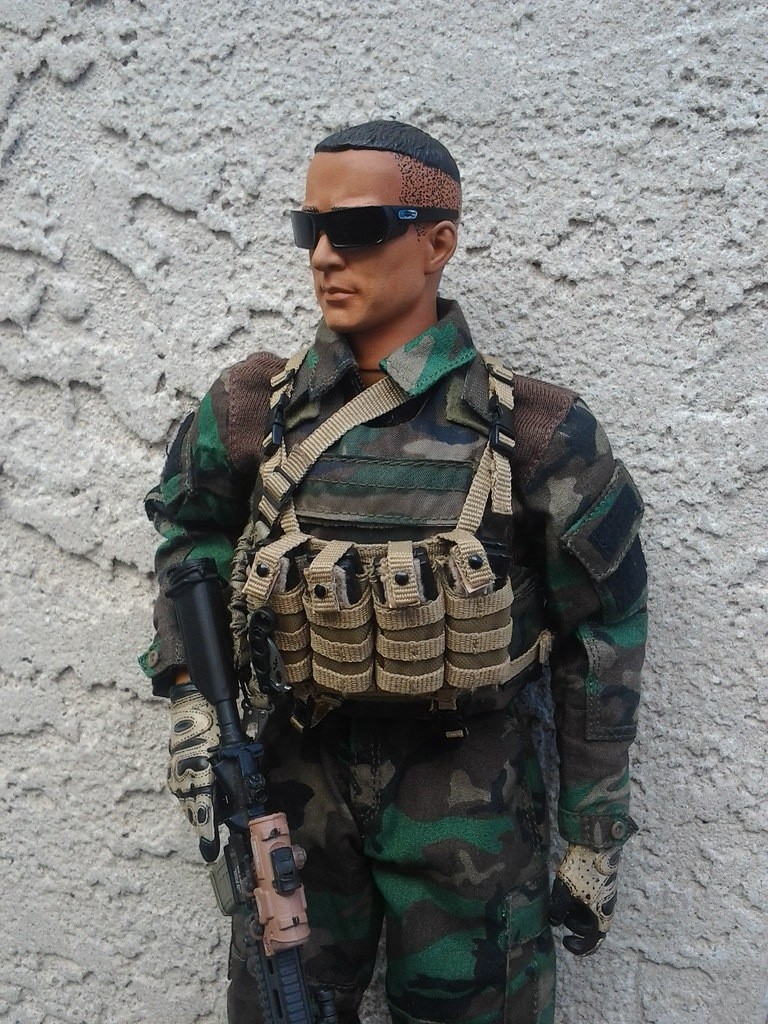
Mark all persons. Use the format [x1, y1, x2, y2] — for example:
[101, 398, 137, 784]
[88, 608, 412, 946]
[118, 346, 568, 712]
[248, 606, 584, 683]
[138, 117, 648, 1024]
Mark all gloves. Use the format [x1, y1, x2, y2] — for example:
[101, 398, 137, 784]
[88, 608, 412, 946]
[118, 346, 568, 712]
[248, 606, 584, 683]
[169, 681, 220, 826]
[549, 842, 619, 957]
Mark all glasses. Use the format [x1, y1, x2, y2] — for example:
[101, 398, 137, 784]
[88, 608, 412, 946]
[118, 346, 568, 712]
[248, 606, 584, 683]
[289, 205, 458, 249]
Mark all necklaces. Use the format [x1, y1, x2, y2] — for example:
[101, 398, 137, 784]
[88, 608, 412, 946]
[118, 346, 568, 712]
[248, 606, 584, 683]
[357, 366, 383, 372]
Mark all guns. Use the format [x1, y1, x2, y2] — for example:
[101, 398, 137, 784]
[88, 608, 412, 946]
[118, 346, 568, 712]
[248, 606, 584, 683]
[154, 556, 344, 1024]
